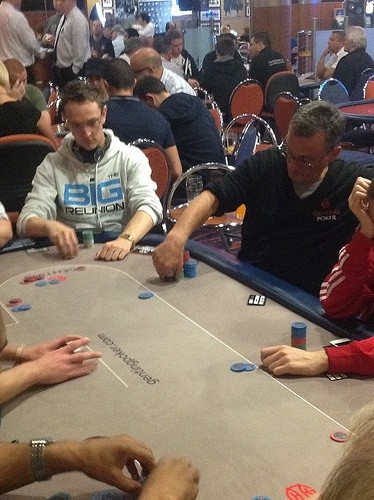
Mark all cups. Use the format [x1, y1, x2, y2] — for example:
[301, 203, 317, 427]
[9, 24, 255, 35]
[225, 134, 237, 153]
[236, 204, 246, 219]
[205, 94, 214, 109]
[292, 66, 297, 75]
[186, 175, 203, 206]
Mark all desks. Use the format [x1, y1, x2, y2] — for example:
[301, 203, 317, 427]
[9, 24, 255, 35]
[0, 232, 374, 500]
[335, 98, 374, 125]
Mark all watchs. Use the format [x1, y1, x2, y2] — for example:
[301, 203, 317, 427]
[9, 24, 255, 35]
[30, 437, 56, 481]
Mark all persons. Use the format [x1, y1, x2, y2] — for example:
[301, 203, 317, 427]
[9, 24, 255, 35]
[135, 77, 226, 197]
[16, 82, 163, 260]
[317, 31, 348, 79]
[249, 32, 287, 87]
[318, 403, 374, 500]
[0, 309, 102, 402]
[153, 103, 374, 297]
[240, 26, 249, 42]
[0, 201, 13, 248]
[99, 59, 182, 178]
[333, 26, 374, 96]
[196, 24, 247, 122]
[0, 0, 200, 146]
[261, 176, 374, 377]
[0, 434, 200, 500]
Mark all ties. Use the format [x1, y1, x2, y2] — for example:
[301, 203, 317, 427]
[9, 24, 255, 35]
[54, 15, 66, 63]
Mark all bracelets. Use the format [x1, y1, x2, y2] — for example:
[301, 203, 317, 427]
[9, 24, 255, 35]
[16, 344, 27, 360]
[119, 233, 135, 251]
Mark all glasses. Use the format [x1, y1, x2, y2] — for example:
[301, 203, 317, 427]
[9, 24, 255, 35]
[65, 111, 102, 133]
[277, 138, 335, 171]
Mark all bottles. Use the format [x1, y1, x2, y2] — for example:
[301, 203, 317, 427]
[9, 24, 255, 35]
[48, 86, 62, 125]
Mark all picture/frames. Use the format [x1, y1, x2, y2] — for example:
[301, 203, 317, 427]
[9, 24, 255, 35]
[198, 0, 221, 38]
[102, 0, 114, 17]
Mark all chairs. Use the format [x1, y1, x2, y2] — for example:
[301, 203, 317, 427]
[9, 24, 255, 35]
[0, 68, 374, 255]
